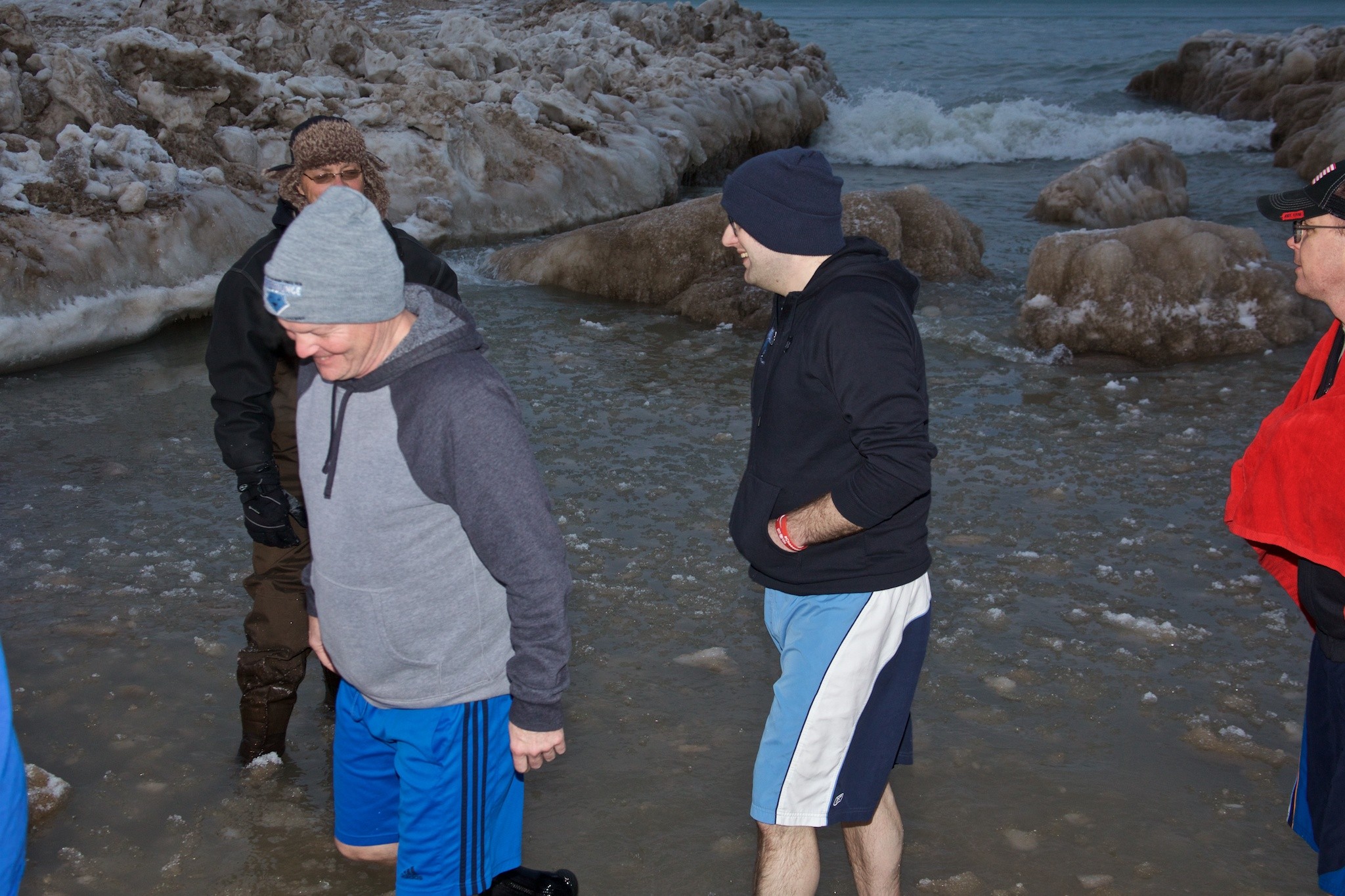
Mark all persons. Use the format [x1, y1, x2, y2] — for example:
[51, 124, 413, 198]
[1222, 158, 1345, 896]
[718, 146, 937, 896]
[205, 115, 462, 765]
[263, 182, 579, 896]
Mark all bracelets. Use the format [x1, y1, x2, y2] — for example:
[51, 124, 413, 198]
[776, 514, 808, 552]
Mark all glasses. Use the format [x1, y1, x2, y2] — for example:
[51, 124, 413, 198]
[728, 215, 741, 237]
[1293, 221, 1345, 244]
[300, 169, 363, 185]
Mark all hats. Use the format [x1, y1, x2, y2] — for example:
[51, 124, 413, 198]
[261, 115, 390, 223]
[1256, 160, 1345, 222]
[720, 146, 847, 256]
[261, 186, 404, 324]
